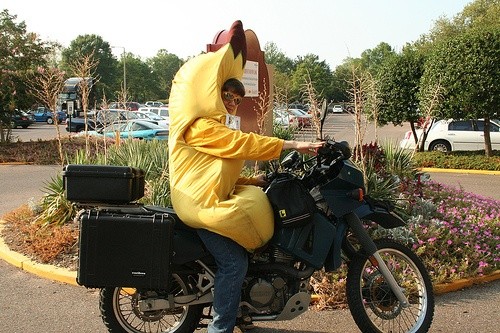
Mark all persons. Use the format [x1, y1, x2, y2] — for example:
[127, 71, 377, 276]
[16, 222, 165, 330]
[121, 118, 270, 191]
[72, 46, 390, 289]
[182, 77, 326, 333]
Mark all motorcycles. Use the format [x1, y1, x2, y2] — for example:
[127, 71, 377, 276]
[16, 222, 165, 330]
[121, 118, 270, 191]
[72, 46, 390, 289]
[60, 100, 436, 333]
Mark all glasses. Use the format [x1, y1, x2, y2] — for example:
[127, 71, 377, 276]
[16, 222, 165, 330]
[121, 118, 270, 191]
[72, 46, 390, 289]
[222, 93, 242, 105]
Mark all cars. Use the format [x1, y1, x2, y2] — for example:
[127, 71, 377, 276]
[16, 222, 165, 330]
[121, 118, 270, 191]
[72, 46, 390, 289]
[3, 77, 347, 140]
[397, 116, 500, 155]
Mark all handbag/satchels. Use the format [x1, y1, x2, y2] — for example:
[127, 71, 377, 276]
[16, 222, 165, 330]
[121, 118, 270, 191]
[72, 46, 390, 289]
[264, 171, 316, 228]
